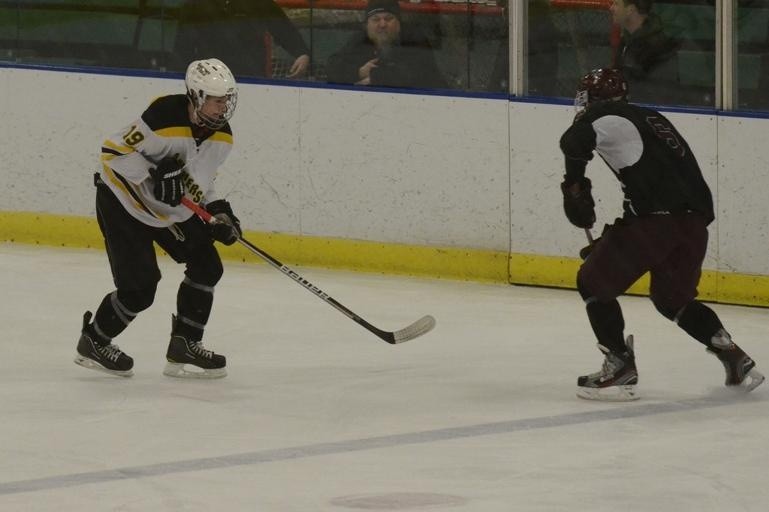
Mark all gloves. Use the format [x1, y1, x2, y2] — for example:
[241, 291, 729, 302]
[563, 175, 597, 226]
[207, 198, 241, 244]
[150, 157, 189, 204]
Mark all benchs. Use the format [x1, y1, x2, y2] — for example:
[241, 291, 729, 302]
[0, 0, 769, 105]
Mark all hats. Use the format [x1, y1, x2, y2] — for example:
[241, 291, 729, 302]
[361, 0, 403, 18]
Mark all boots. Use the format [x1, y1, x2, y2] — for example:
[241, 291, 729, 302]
[577, 336, 639, 388]
[711, 326, 757, 388]
[77, 313, 133, 370]
[167, 313, 226, 366]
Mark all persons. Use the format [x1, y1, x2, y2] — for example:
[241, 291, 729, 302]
[486, 0, 582, 100]
[325, 0, 449, 89]
[171, 0, 316, 83]
[559, 66, 766, 388]
[73, 58, 242, 372]
[609, 0, 698, 108]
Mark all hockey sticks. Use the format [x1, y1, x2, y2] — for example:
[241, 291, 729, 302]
[181, 196, 436, 345]
[584, 227, 633, 352]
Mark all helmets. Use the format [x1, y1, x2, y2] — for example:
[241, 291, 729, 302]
[573, 69, 628, 109]
[186, 57, 238, 129]
